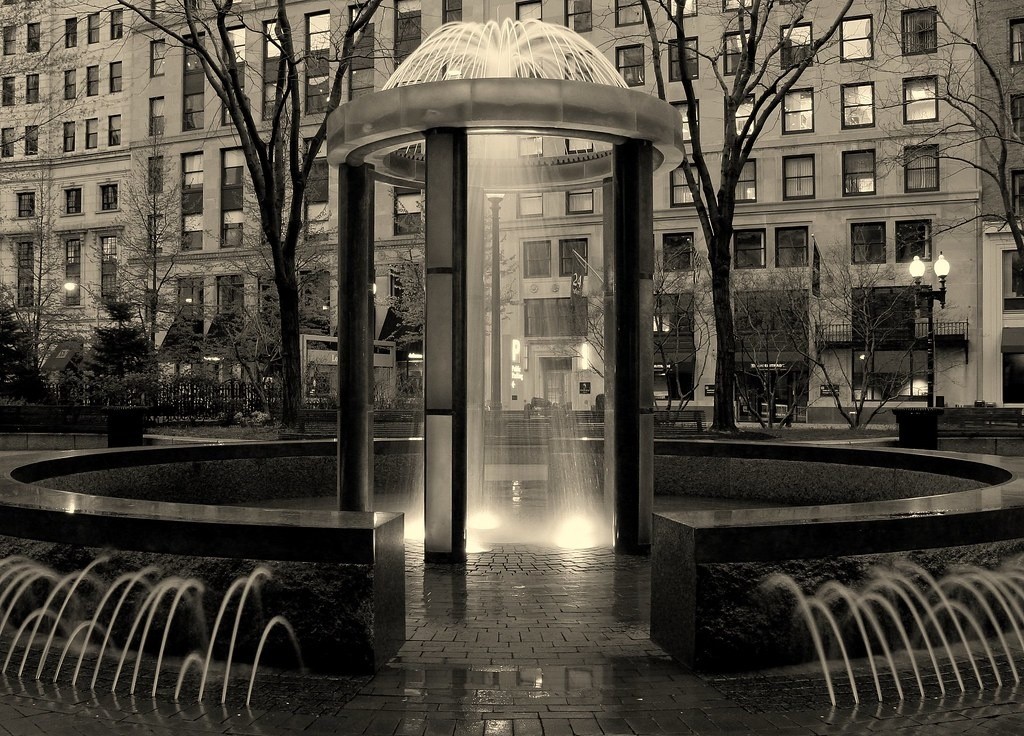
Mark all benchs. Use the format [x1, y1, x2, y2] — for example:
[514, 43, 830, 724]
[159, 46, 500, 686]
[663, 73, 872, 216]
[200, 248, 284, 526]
[939, 407, 1024, 455]
[484, 408, 557, 442]
[284, 408, 416, 445]
[655, 411, 713, 438]
[565, 409, 604, 440]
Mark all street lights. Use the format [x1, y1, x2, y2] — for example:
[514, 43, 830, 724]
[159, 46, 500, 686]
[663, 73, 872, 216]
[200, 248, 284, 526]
[910, 251, 950, 406]
